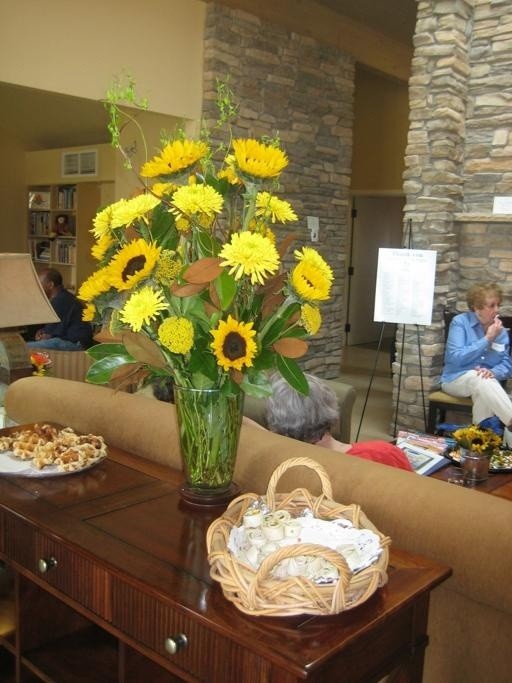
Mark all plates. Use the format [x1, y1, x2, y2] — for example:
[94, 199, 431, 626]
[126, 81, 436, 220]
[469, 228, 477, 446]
[0, 451, 108, 477]
[445, 447, 512, 474]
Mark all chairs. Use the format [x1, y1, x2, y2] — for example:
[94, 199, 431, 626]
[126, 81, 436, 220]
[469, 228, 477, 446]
[426, 311, 512, 435]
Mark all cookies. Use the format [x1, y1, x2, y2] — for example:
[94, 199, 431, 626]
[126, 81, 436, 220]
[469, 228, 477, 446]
[0, 423, 109, 473]
[242, 507, 359, 580]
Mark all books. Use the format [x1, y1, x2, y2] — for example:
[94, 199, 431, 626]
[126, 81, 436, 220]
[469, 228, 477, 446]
[25, 186, 76, 262]
[392, 429, 457, 475]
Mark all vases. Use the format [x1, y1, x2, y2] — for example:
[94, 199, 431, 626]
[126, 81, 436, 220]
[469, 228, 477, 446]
[173, 385, 245, 515]
[464, 454, 484, 481]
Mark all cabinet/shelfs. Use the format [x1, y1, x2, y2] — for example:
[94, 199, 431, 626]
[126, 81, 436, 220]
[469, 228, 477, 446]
[24, 181, 99, 297]
[0, 419, 456, 683]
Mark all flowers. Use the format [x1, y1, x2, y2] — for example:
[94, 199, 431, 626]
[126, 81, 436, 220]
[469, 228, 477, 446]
[78, 68, 339, 483]
[452, 423, 503, 457]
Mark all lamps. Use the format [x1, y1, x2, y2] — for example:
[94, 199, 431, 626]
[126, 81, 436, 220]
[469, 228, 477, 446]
[0, 252, 61, 384]
[355, 216, 437, 441]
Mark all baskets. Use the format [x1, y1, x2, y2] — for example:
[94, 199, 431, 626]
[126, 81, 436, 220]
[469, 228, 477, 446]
[206, 457, 392, 617]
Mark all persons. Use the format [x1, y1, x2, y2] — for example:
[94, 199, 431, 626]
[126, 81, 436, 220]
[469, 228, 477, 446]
[265, 370, 413, 471]
[23, 267, 95, 351]
[440, 279, 511, 435]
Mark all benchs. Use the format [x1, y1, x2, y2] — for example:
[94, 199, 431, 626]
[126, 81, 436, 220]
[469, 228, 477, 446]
[252, 368, 356, 442]
